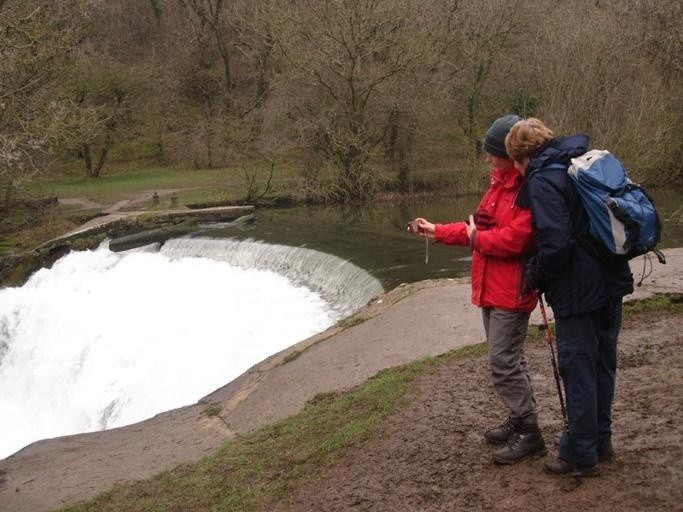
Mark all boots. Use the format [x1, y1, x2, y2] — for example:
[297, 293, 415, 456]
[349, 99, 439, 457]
[485, 414, 545, 464]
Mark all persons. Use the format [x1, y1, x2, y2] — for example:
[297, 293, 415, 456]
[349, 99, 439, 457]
[405, 114, 548, 466]
[505, 117, 635, 476]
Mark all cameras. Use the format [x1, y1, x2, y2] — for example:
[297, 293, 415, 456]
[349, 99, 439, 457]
[406, 220, 422, 233]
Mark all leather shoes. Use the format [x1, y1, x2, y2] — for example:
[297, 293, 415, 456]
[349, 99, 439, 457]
[544, 441, 613, 477]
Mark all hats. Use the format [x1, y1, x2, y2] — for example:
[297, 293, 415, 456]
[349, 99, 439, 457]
[483, 115, 522, 159]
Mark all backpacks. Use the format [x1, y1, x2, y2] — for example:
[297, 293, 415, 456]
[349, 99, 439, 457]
[528, 149, 667, 287]
